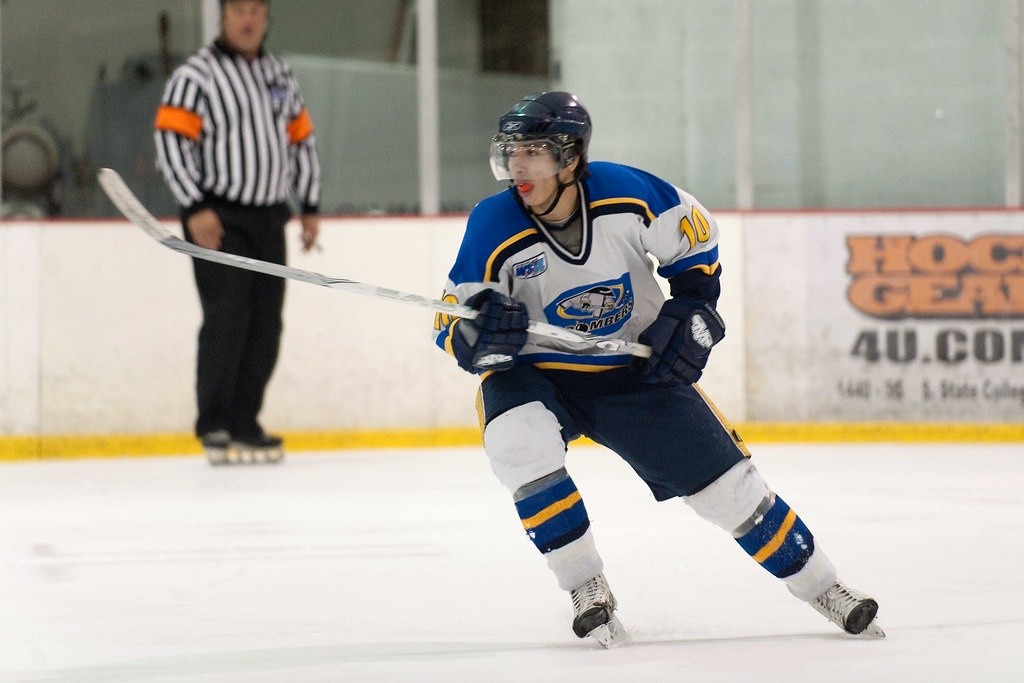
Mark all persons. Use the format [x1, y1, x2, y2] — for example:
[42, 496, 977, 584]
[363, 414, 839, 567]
[152, 0, 327, 453]
[431, 89, 880, 642]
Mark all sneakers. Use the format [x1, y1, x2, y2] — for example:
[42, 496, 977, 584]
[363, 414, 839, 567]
[569, 572, 632, 649]
[197, 421, 286, 466]
[812, 580, 887, 642]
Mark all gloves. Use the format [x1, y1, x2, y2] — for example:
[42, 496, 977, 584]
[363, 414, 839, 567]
[639, 297, 724, 389]
[451, 290, 528, 377]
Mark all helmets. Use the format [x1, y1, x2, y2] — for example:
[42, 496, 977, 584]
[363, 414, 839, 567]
[486, 91, 592, 184]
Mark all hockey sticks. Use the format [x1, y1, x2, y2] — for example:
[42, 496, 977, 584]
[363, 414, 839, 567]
[95, 166, 652, 358]
